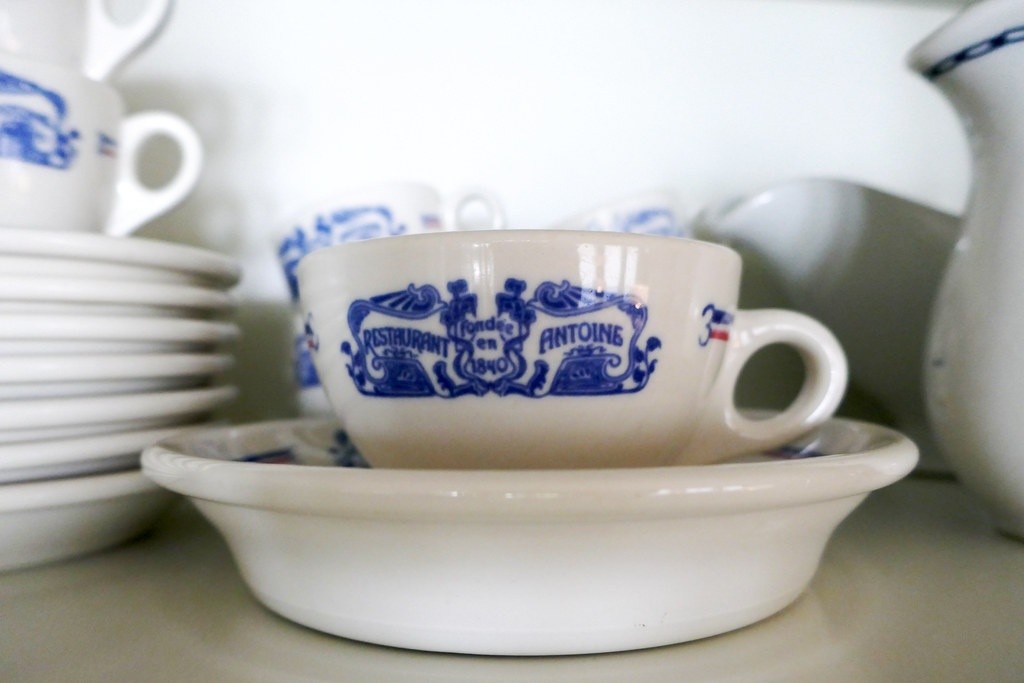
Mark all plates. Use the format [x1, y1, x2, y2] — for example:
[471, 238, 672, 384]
[0, 230, 243, 571]
[141, 411, 920, 658]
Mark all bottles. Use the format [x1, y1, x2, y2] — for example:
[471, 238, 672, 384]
[909, 0, 1023, 538]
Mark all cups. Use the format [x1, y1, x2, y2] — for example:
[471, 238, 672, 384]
[0, 58, 202, 237]
[279, 200, 508, 419]
[0, 1, 176, 82]
[299, 228, 847, 468]
